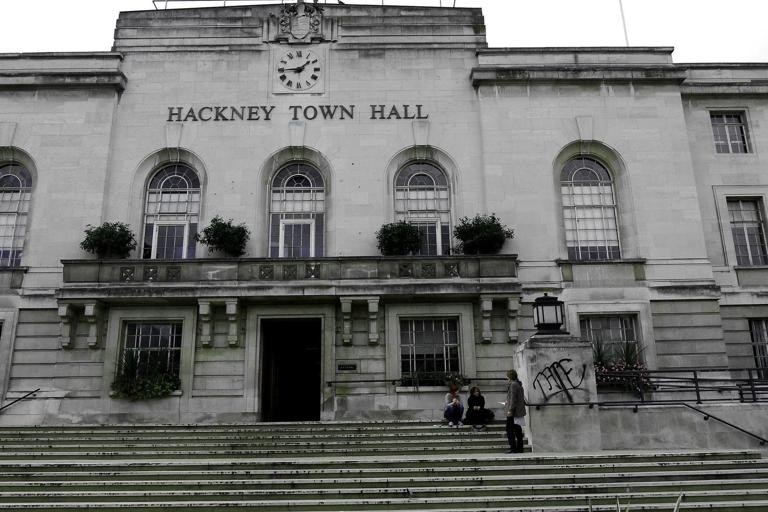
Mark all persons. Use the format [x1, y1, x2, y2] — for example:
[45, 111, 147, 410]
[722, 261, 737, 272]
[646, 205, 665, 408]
[443, 385, 485, 426]
[505, 369, 527, 453]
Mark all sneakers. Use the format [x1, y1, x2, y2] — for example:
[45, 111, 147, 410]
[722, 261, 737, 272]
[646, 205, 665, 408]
[449, 421, 464, 426]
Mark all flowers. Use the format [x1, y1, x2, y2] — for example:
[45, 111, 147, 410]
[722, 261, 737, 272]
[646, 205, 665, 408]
[593, 359, 654, 393]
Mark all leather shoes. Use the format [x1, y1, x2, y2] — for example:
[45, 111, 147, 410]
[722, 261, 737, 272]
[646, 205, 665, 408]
[505, 446, 523, 453]
